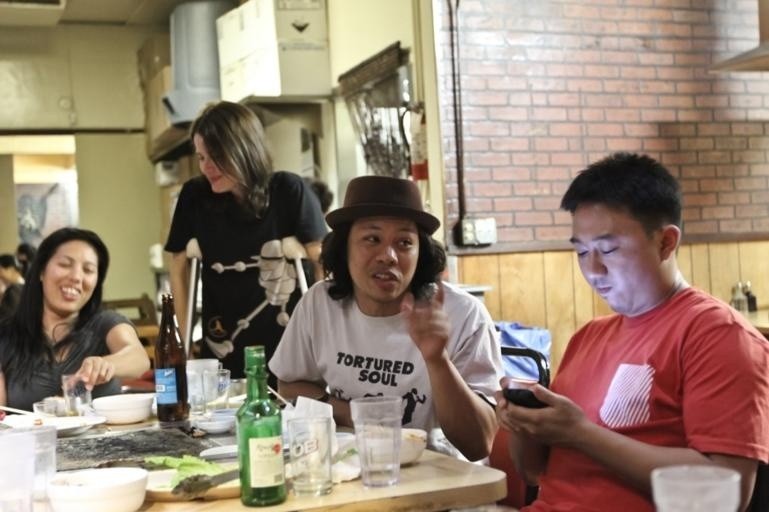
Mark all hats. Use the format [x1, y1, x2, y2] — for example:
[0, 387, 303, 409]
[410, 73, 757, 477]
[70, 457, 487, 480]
[325, 175, 440, 235]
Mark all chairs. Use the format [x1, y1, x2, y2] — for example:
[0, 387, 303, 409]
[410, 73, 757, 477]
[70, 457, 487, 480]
[104, 297, 158, 362]
[484, 346, 549, 511]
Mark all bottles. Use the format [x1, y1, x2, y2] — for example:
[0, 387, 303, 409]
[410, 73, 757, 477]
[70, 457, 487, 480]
[730, 281, 757, 313]
[154, 294, 190, 425]
[238, 344, 287, 507]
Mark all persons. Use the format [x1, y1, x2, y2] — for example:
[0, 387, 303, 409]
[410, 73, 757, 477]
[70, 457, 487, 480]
[0, 242, 36, 314]
[267, 174, 503, 461]
[498, 155, 768, 511]
[164, 102, 330, 399]
[0, 228, 152, 415]
[302, 181, 332, 284]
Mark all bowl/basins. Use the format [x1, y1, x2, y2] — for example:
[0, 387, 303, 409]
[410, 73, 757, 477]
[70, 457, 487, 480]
[93, 394, 154, 426]
[374, 428, 428, 467]
[43, 466, 149, 512]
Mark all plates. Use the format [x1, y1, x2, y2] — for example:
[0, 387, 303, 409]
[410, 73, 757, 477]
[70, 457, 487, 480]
[3, 412, 106, 437]
[144, 461, 241, 501]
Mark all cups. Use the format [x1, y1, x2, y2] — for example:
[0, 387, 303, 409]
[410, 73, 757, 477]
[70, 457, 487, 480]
[650, 464, 742, 511]
[0, 424, 58, 512]
[185, 358, 403, 497]
[32, 374, 92, 416]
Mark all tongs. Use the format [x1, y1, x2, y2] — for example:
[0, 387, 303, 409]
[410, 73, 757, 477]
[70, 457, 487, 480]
[96, 448, 291, 501]
